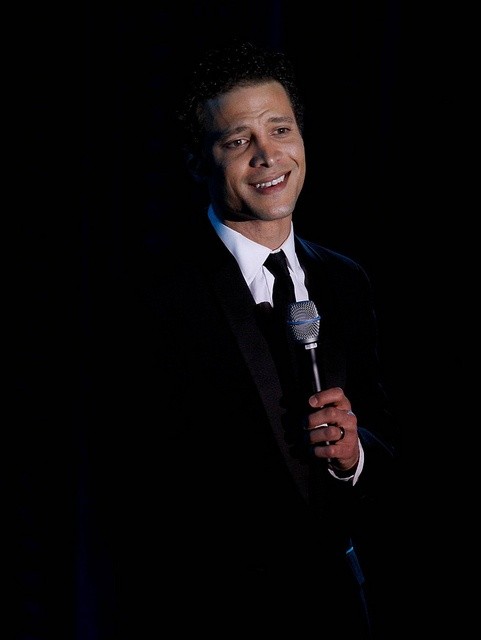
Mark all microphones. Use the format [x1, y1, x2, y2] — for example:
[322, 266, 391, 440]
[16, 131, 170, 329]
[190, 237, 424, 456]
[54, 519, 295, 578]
[287, 299, 336, 448]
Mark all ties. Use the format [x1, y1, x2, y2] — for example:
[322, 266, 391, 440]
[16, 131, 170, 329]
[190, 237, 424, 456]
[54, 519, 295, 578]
[263, 252, 308, 405]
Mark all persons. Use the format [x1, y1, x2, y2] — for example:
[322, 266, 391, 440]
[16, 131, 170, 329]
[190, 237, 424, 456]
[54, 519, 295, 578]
[128, 40, 418, 590]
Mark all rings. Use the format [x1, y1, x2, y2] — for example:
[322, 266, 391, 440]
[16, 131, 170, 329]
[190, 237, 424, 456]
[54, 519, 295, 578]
[337, 426, 346, 443]
